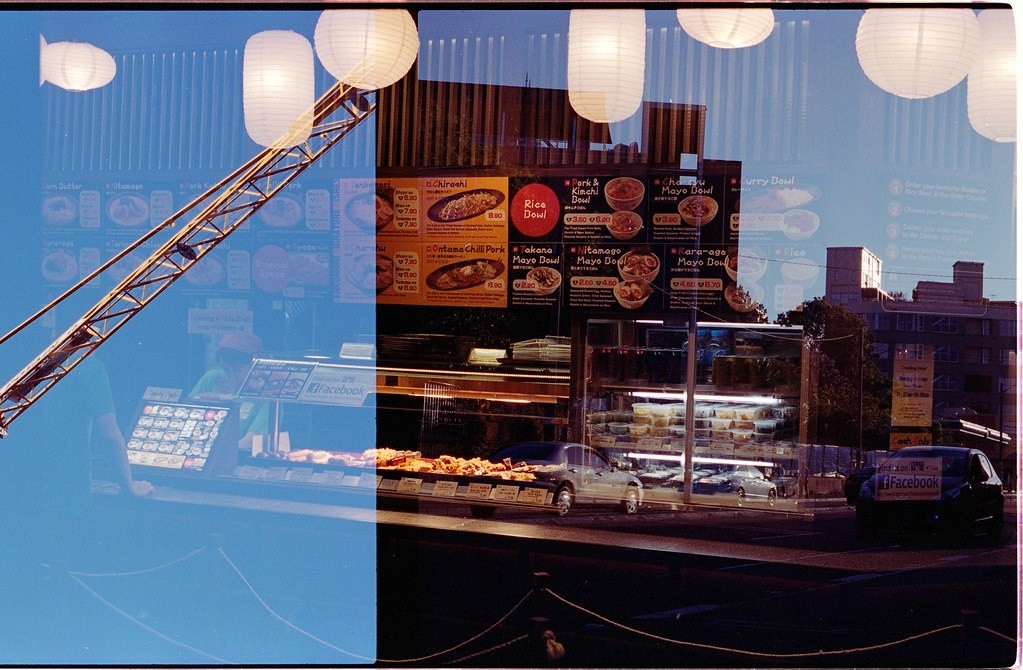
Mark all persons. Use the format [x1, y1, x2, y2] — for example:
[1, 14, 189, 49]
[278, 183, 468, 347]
[179, 332, 287, 461]
[26, 305, 155, 606]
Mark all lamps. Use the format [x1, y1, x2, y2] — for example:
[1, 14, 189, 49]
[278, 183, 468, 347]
[855, 7, 976, 99]
[676, 7, 774, 50]
[965, 9, 1016, 144]
[567, 8, 648, 123]
[38, 33, 116, 94]
[241, 29, 316, 150]
[313, 9, 421, 92]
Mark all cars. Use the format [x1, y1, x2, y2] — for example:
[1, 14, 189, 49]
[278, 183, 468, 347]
[492, 441, 643, 514]
[856, 445, 1003, 537]
[609, 452, 798, 508]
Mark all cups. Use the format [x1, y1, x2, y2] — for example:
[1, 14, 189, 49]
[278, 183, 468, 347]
[586, 344, 683, 388]
[586, 401, 798, 493]
[712, 355, 801, 391]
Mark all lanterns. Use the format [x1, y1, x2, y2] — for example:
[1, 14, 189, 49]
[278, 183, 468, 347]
[240, 29, 315, 151]
[674, 7, 777, 52]
[968, 8, 1016, 144]
[566, 7, 646, 125]
[38, 30, 51, 88]
[855, 6, 979, 99]
[310, 8, 418, 93]
[44, 39, 118, 96]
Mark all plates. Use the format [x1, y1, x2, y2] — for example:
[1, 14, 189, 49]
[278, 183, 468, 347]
[495, 357, 571, 366]
[351, 329, 480, 367]
[426, 258, 504, 291]
[426, 187, 505, 222]
[734, 184, 823, 212]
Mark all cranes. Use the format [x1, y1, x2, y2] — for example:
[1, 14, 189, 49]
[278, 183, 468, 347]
[0, 48, 376, 452]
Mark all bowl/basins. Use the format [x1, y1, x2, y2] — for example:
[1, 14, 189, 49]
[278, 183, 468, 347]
[613, 280, 652, 309]
[127, 406, 227, 467]
[38, 247, 81, 284]
[103, 251, 147, 285]
[345, 192, 399, 233]
[526, 267, 562, 294]
[344, 253, 398, 299]
[606, 212, 643, 240]
[244, 372, 305, 397]
[105, 192, 151, 230]
[724, 249, 767, 283]
[256, 192, 306, 231]
[38, 191, 80, 226]
[780, 258, 820, 289]
[179, 252, 227, 287]
[678, 196, 718, 226]
[605, 177, 644, 211]
[178, 193, 224, 230]
[725, 280, 763, 312]
[617, 251, 660, 284]
[779, 209, 821, 240]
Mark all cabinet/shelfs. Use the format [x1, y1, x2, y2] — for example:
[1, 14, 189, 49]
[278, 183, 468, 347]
[235, 355, 573, 516]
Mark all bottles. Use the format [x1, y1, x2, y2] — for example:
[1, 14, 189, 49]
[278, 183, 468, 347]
[733, 331, 763, 358]
[684, 329, 731, 386]
